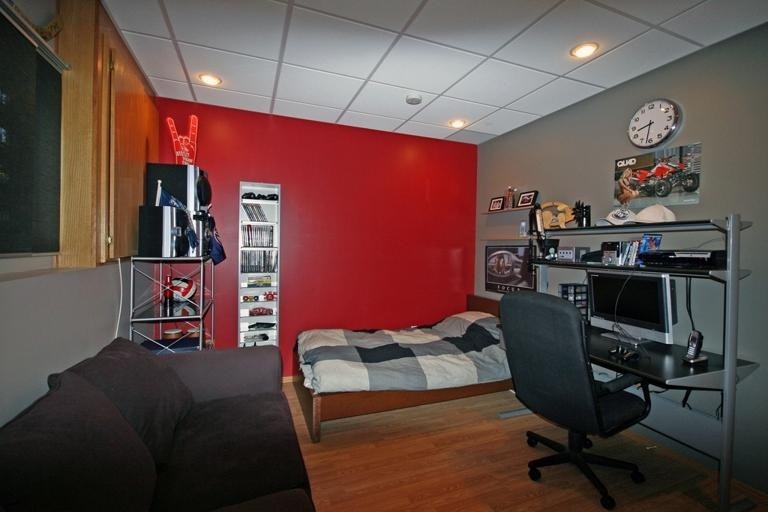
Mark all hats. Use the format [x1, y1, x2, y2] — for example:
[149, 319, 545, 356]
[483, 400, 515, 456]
[599, 208, 636, 226]
[622, 204, 676, 224]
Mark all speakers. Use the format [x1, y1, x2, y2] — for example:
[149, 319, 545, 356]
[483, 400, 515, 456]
[137, 205, 187, 258]
[144, 162, 211, 258]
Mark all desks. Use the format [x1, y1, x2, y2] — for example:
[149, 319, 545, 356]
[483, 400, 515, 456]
[499, 215, 758, 510]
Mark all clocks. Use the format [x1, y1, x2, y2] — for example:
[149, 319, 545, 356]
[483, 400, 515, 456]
[627, 97, 680, 149]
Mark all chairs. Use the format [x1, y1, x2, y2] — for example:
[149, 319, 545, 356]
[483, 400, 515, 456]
[501, 289, 653, 511]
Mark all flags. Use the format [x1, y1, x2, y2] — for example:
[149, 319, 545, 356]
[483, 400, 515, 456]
[153, 186, 199, 249]
[209, 218, 227, 266]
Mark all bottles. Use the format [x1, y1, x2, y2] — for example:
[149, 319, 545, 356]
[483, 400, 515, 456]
[163, 275, 174, 317]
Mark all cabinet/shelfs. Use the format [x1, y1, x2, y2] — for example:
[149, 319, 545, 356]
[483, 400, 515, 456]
[129, 256, 215, 353]
[236, 179, 281, 348]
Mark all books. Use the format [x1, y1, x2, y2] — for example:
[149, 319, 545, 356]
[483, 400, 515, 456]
[640, 233, 663, 252]
[241, 203, 279, 273]
[601, 241, 642, 266]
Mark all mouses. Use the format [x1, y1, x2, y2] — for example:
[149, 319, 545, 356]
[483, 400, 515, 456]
[609, 344, 625, 355]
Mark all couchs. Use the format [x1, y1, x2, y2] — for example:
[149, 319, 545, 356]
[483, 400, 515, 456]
[1, 344, 318, 511]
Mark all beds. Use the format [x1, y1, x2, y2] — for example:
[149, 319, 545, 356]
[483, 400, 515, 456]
[292, 292, 514, 443]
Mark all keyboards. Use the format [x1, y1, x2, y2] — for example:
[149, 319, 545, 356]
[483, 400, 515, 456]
[591, 363, 624, 384]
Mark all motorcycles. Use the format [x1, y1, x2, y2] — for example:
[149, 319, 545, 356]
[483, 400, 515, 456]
[626, 153, 700, 196]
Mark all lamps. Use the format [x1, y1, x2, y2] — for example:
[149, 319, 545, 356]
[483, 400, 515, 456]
[525, 202, 547, 275]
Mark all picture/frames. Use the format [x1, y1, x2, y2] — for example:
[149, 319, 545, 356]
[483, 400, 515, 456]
[488, 190, 539, 212]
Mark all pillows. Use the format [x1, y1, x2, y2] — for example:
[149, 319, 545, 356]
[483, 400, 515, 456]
[1, 336, 196, 511]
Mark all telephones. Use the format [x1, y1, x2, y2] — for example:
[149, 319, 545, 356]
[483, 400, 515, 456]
[681, 330, 708, 364]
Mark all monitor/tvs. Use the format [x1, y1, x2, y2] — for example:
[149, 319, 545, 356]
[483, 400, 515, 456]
[586, 268, 678, 347]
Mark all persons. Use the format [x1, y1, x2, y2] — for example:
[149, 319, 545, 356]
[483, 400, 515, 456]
[493, 255, 507, 275]
[618, 168, 640, 207]
[649, 236, 657, 250]
